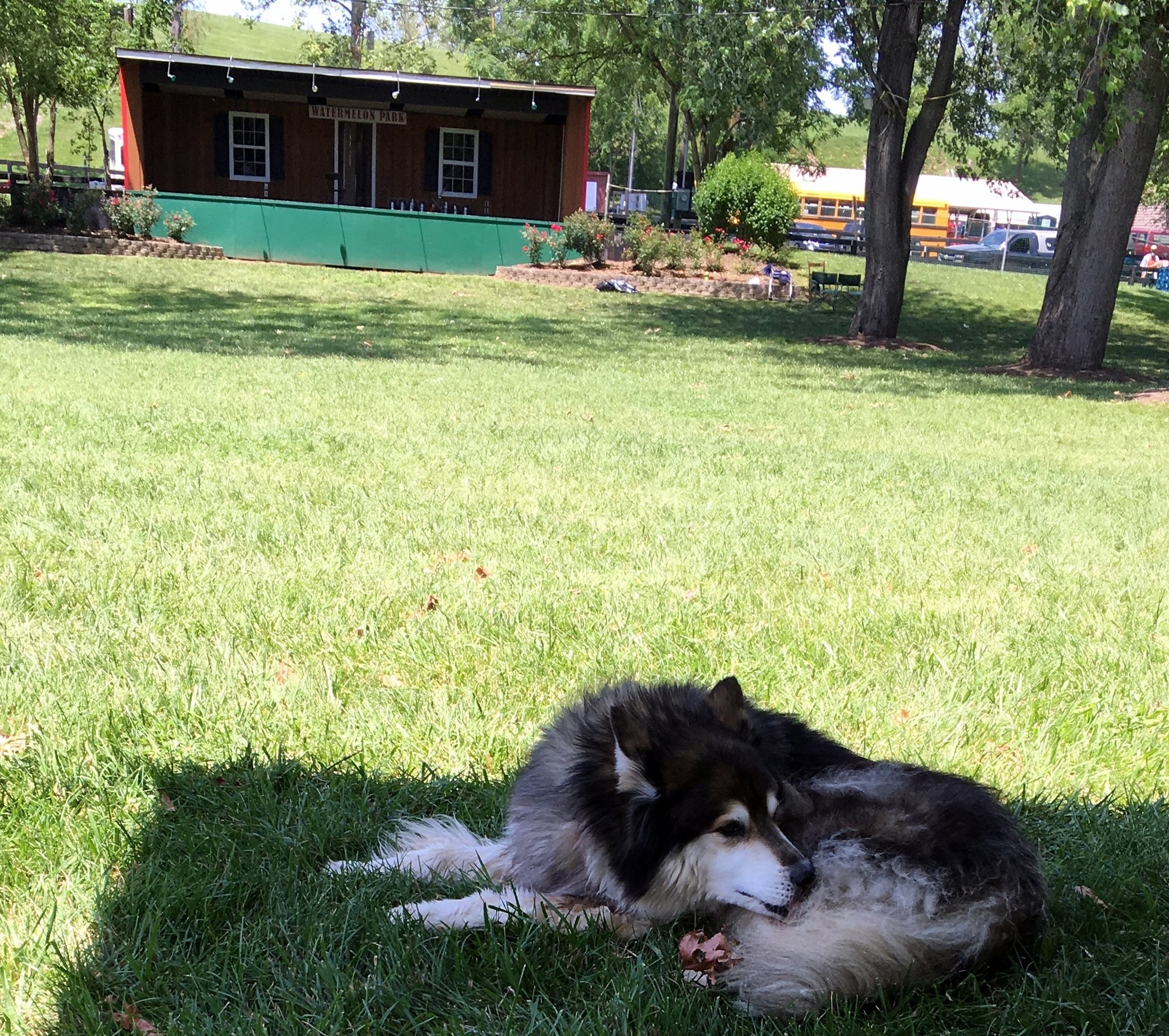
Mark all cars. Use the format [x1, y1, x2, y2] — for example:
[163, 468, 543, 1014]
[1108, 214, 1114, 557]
[782, 222, 839, 252]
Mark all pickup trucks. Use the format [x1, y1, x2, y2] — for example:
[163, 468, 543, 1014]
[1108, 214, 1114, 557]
[937, 230, 1059, 268]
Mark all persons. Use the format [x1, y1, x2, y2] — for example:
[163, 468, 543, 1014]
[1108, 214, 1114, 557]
[960, 226, 965, 235]
[1140, 245, 1159, 288]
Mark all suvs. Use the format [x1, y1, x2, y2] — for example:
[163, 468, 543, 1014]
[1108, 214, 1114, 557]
[835, 219, 922, 256]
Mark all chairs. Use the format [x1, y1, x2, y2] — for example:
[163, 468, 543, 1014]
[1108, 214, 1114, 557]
[807, 260, 862, 313]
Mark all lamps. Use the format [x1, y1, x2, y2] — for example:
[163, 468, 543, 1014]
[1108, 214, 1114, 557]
[312, 63, 317, 92]
[531, 79, 538, 109]
[167, 53, 175, 81]
[392, 70, 400, 99]
[226, 55, 234, 82]
[475, 75, 481, 102]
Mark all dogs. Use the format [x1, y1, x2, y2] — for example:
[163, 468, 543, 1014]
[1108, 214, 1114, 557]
[320, 676, 1052, 1017]
